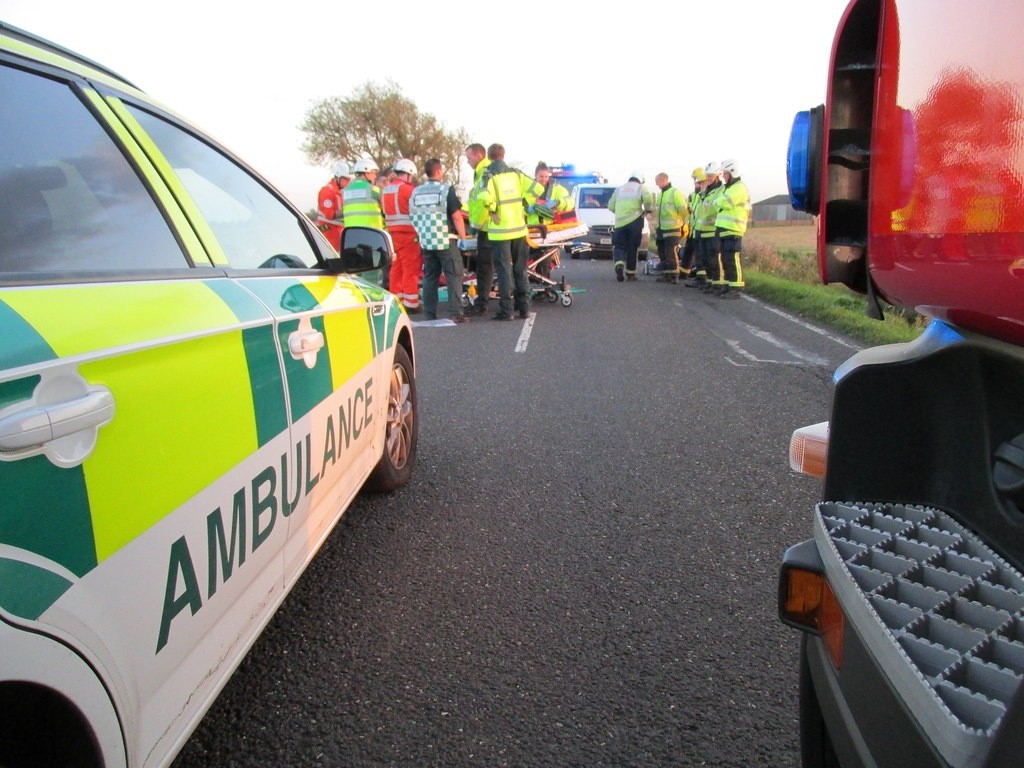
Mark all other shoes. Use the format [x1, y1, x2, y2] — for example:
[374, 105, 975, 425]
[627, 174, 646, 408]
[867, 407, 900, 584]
[628, 275, 637, 281]
[491, 312, 514, 321]
[405, 307, 421, 314]
[656, 273, 678, 283]
[679, 271, 743, 299]
[453, 315, 471, 323]
[520, 310, 531, 318]
[466, 305, 488, 316]
[615, 264, 624, 282]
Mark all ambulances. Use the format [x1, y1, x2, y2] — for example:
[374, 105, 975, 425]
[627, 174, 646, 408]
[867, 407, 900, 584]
[1, 25, 420, 768]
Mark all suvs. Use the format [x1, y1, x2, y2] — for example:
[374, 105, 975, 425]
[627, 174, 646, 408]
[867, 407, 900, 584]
[572, 185, 654, 262]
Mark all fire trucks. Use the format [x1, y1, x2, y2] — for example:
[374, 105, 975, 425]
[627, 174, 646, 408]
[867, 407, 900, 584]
[780, 2, 1024, 766]
[549, 163, 608, 203]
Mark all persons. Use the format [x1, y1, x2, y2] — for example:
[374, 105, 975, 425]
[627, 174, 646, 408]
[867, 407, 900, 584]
[318, 160, 422, 314]
[464, 144, 575, 321]
[408, 158, 470, 323]
[584, 195, 600, 206]
[608, 160, 751, 299]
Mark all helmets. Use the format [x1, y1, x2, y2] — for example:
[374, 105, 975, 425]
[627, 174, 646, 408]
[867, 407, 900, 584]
[695, 175, 706, 182]
[334, 162, 353, 178]
[353, 158, 380, 173]
[720, 159, 742, 178]
[704, 162, 722, 175]
[394, 159, 417, 176]
[692, 168, 703, 177]
[628, 174, 646, 184]
